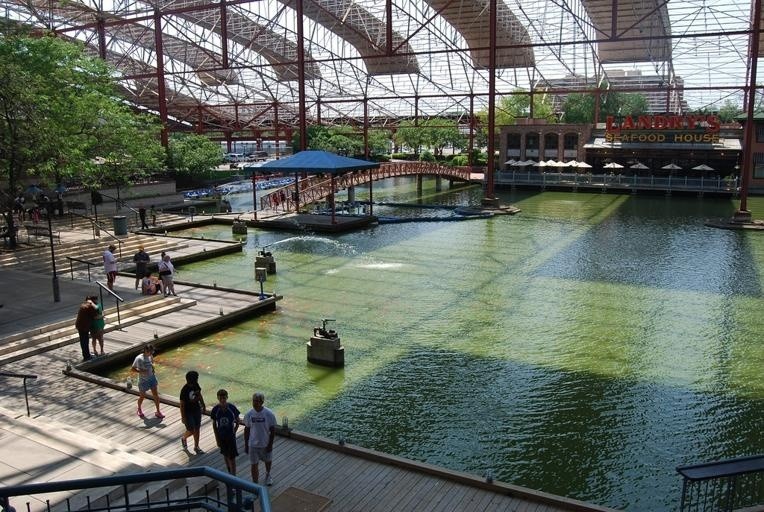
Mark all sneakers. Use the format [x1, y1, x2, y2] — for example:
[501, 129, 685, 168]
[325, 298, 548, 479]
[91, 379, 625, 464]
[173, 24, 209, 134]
[264, 472, 274, 486]
[155, 411, 165, 419]
[193, 446, 204, 454]
[137, 410, 144, 417]
[181, 436, 187, 450]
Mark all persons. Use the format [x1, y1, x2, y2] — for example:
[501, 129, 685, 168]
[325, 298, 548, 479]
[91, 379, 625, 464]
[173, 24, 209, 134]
[241, 393, 277, 487]
[91, 295, 104, 356]
[210, 389, 241, 484]
[13, 185, 67, 225]
[132, 246, 178, 297]
[74, 295, 105, 361]
[272, 192, 278, 211]
[149, 204, 157, 225]
[279, 190, 286, 210]
[179, 371, 206, 454]
[139, 203, 147, 229]
[102, 244, 119, 294]
[129, 344, 166, 419]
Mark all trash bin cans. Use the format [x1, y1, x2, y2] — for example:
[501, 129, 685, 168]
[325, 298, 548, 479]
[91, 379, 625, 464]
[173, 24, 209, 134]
[114, 216, 128, 234]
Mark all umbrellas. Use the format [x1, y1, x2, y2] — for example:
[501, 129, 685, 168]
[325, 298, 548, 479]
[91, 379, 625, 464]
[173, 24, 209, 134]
[503, 156, 716, 174]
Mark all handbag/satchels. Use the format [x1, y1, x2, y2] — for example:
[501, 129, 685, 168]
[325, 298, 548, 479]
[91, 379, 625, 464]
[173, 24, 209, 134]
[159, 263, 172, 277]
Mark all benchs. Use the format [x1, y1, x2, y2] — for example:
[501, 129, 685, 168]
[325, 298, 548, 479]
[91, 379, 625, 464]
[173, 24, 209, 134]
[24, 224, 62, 245]
